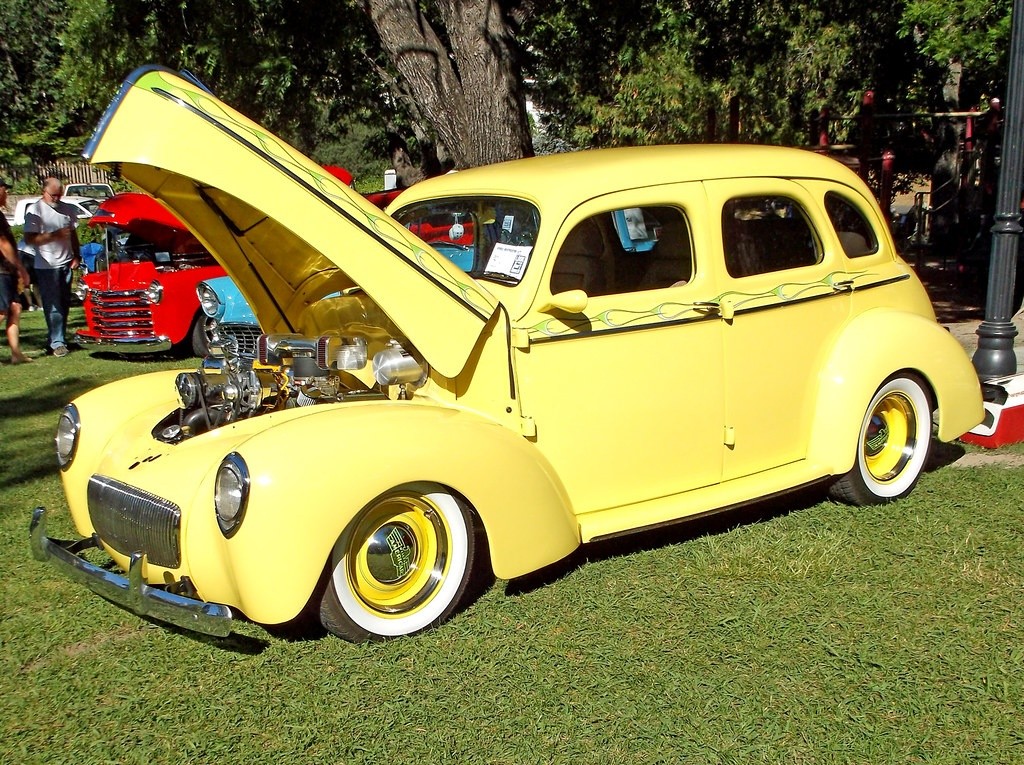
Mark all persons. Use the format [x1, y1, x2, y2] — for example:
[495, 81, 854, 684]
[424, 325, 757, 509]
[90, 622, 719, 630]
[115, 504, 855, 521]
[0, 176, 81, 365]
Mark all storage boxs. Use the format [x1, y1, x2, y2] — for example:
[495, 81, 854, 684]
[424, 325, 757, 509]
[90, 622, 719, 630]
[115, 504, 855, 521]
[958, 372, 1024, 448]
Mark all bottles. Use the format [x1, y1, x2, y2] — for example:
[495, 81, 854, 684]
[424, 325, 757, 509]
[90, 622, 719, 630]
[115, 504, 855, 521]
[63, 216, 70, 227]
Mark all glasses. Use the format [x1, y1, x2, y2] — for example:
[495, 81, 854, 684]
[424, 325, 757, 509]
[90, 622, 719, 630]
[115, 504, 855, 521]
[45, 190, 62, 199]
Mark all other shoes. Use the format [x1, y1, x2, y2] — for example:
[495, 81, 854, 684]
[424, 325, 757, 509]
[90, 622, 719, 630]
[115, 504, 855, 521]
[29, 306, 35, 312]
[37, 307, 45, 312]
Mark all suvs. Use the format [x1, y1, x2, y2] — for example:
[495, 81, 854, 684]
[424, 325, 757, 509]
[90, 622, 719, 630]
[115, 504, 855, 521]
[24, 63, 984, 647]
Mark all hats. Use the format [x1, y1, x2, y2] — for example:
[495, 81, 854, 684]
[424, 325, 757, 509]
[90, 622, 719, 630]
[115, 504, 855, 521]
[0, 178, 13, 188]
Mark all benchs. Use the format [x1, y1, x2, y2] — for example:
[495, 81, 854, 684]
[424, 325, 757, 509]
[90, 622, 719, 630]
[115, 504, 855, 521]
[744, 215, 867, 270]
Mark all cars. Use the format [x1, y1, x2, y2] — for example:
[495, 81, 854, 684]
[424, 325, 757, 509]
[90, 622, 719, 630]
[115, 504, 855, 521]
[194, 204, 656, 360]
[70, 163, 479, 355]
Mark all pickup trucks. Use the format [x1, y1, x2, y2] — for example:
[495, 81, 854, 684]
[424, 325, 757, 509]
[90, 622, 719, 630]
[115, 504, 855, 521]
[14, 183, 116, 226]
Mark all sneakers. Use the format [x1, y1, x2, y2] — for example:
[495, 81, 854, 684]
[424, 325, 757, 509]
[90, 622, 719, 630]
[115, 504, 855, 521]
[54, 345, 68, 357]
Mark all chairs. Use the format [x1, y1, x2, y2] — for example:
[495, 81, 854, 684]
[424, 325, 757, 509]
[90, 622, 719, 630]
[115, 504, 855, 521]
[549, 213, 623, 297]
[634, 213, 760, 290]
